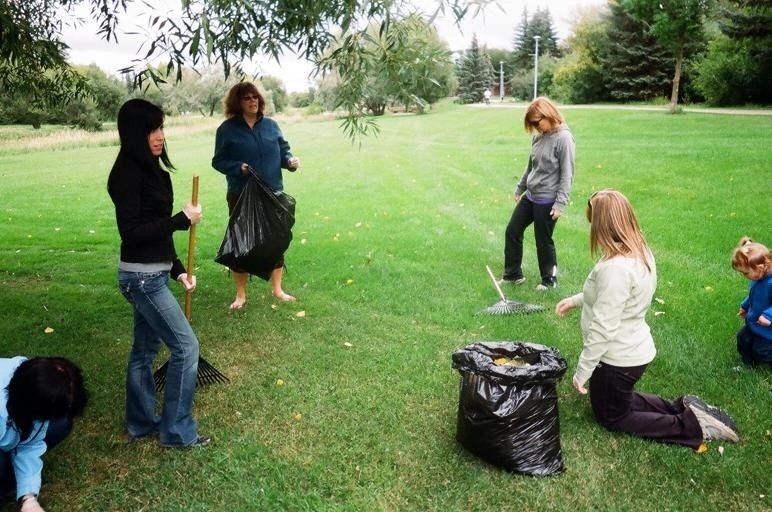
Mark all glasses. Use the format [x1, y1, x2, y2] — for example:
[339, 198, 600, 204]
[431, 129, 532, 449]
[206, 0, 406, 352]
[240, 95, 259, 101]
[587, 191, 598, 209]
[531, 118, 542, 127]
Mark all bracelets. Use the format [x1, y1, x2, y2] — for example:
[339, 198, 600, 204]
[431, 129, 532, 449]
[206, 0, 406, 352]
[21, 496, 37, 503]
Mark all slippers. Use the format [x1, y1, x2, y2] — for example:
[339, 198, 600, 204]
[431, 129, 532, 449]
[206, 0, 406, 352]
[229, 299, 246, 311]
[272, 293, 296, 302]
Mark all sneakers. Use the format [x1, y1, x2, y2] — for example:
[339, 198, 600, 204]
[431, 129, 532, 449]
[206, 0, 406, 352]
[689, 404, 739, 444]
[682, 394, 735, 431]
[497, 276, 525, 285]
[536, 276, 558, 291]
[159, 434, 210, 448]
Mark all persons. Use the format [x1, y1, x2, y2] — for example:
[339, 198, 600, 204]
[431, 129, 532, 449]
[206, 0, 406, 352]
[211, 82, 300, 309]
[731, 236, 772, 364]
[483, 88, 491, 105]
[106, 98, 211, 448]
[496, 97, 576, 291]
[556, 189, 740, 450]
[0, 355, 88, 512]
